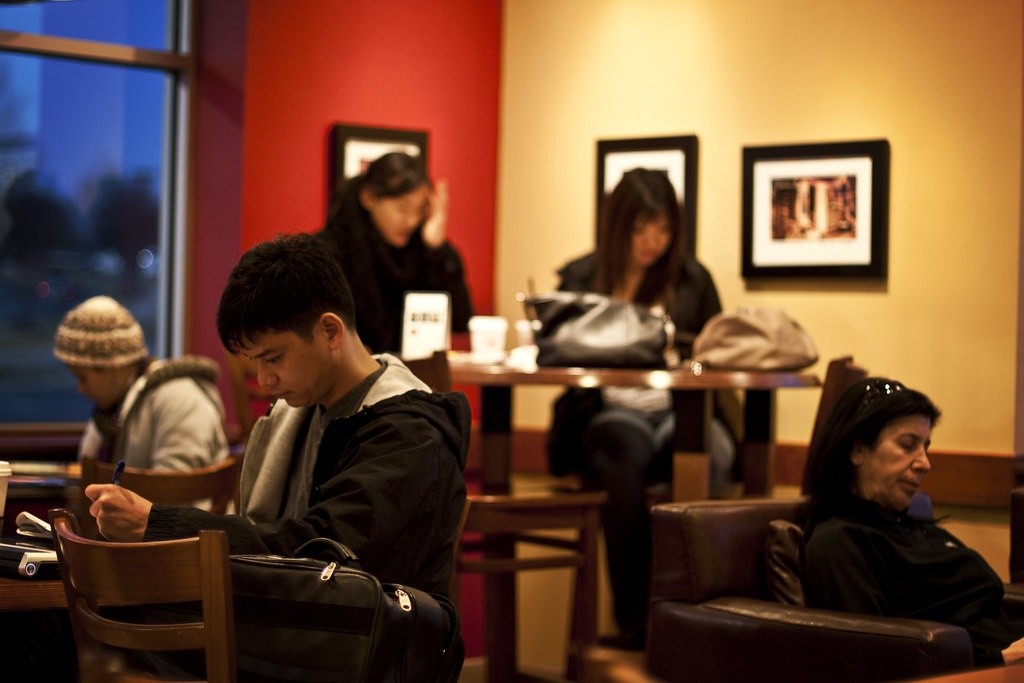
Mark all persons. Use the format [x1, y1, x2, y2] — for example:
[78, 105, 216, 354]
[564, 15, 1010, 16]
[543, 170, 732, 650]
[317, 153, 478, 393]
[801, 378, 1024, 668]
[53, 295, 235, 515]
[86, 232, 470, 593]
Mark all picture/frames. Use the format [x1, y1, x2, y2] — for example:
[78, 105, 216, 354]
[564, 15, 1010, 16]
[592, 134, 700, 260]
[328, 123, 429, 227]
[742, 139, 892, 285]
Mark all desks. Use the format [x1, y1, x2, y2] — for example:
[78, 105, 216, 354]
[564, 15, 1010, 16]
[449, 353, 821, 683]
[3, 475, 76, 541]
[0, 574, 150, 683]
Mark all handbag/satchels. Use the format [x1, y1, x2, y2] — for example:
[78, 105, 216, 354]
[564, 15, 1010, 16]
[692, 308, 821, 373]
[228, 533, 467, 681]
[522, 293, 666, 372]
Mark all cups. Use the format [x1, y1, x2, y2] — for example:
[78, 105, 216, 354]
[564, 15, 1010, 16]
[466, 314, 507, 363]
[516, 318, 535, 346]
[0, 461, 12, 518]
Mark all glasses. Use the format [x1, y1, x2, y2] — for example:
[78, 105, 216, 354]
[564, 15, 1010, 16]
[856, 379, 906, 421]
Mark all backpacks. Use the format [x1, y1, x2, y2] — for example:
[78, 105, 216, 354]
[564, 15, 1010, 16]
[888, 523, 1005, 618]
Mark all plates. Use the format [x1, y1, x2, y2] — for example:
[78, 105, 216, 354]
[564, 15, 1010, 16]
[15, 528, 53, 539]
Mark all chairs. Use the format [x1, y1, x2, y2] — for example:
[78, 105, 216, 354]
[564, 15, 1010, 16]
[49, 451, 1024, 683]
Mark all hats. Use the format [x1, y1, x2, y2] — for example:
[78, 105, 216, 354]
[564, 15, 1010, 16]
[52, 295, 148, 367]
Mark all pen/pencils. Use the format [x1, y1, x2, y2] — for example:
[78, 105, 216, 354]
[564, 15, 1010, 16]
[113, 461, 125, 484]
